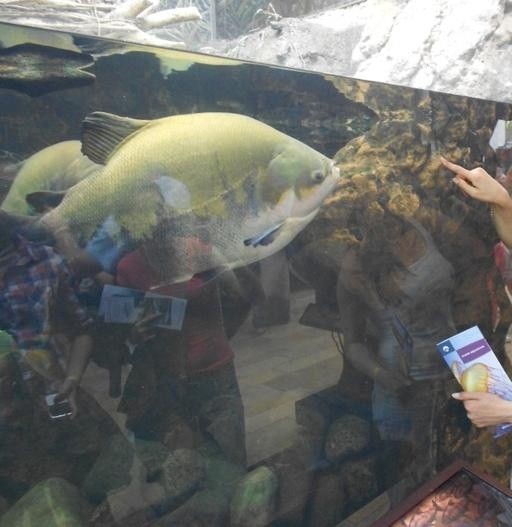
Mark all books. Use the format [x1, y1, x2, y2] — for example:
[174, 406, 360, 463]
[435, 323, 511, 438]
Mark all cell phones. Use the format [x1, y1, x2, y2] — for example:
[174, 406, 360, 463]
[44, 391, 73, 421]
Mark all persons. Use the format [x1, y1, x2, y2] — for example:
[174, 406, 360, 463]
[438, 153, 512, 429]
[0, 161, 497, 526]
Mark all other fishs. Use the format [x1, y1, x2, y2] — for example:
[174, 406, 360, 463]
[0, 139, 107, 217]
[0, 110, 342, 289]
